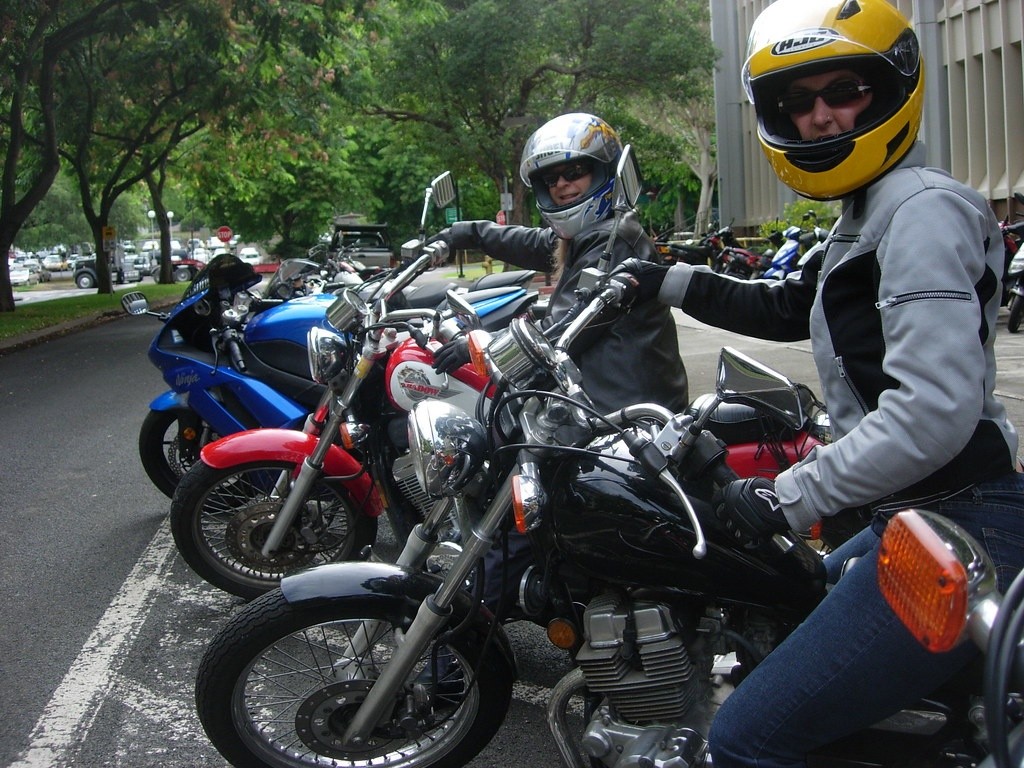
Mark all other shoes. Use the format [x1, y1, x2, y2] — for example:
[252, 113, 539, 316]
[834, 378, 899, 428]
[413, 646, 464, 715]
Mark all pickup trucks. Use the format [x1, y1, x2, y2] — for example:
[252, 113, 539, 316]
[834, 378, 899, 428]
[330, 230, 397, 280]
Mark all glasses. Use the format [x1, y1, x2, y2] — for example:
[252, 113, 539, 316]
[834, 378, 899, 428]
[543, 164, 593, 187]
[777, 78, 873, 113]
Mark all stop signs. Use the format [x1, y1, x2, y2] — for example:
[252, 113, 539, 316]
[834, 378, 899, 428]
[496, 210, 506, 225]
[216, 226, 233, 242]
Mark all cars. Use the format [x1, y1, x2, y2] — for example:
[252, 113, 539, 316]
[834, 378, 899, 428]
[9, 233, 263, 290]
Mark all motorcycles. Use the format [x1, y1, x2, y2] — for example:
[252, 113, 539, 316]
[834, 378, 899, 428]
[119, 145, 1024, 767]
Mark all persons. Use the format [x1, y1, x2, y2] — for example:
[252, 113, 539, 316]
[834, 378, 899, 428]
[431, 114, 688, 611]
[609, 1, 1024, 768]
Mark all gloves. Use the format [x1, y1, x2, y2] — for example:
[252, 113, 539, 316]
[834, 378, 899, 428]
[425, 226, 456, 258]
[432, 335, 472, 375]
[608, 257, 671, 301]
[712, 477, 792, 546]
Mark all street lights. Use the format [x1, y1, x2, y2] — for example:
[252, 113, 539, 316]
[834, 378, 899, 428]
[148, 209, 156, 259]
[167, 211, 174, 241]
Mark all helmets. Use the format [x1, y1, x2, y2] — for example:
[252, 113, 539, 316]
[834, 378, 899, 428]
[519, 113, 624, 240]
[740, 0, 925, 201]
